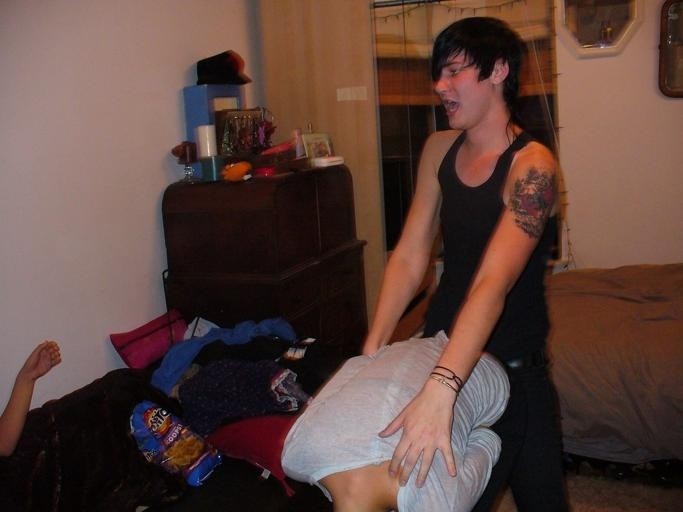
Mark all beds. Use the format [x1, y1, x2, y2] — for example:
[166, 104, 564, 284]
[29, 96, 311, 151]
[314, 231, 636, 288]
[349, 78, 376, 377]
[386, 223, 683, 485]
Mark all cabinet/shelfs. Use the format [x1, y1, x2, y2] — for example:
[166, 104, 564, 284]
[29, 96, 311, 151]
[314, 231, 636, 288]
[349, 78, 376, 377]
[162, 164, 367, 372]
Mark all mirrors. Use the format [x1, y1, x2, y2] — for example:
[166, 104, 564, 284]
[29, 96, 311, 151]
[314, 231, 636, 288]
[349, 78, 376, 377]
[555, 0, 644, 59]
[657, 0, 683, 98]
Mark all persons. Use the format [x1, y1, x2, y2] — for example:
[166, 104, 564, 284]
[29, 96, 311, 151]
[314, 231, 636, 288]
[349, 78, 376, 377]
[266, 325, 509, 510]
[0, 333, 61, 460]
[349, 13, 574, 512]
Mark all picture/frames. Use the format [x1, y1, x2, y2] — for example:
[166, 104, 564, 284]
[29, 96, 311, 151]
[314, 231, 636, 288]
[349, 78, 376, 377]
[301, 134, 332, 158]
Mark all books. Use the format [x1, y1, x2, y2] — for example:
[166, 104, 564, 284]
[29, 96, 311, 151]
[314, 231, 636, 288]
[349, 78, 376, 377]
[300, 130, 332, 168]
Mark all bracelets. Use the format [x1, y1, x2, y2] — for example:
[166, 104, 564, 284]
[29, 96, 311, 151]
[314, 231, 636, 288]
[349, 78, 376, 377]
[429, 364, 462, 397]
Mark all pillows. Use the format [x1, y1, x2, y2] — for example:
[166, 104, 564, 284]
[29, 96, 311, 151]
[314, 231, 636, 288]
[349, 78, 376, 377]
[110, 307, 188, 368]
